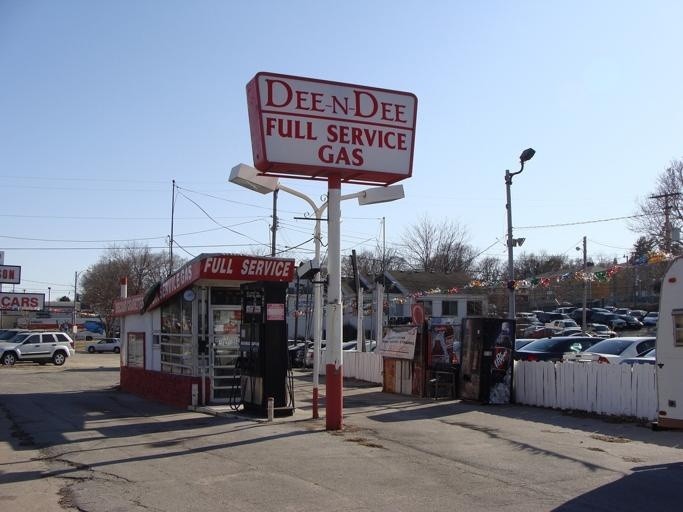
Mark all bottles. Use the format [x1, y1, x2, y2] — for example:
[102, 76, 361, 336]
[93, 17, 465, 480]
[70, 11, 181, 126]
[432, 339, 444, 360]
[492, 321, 512, 380]
[470, 328, 482, 372]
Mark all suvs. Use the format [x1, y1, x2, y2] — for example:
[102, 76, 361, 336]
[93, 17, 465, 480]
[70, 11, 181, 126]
[0, 332, 74, 366]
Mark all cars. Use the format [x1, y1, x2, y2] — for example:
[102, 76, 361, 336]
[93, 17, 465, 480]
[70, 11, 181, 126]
[513, 338, 535, 350]
[512, 304, 657, 337]
[85, 337, 121, 353]
[513, 336, 605, 363]
[286, 332, 376, 369]
[568, 336, 656, 364]
[0, 327, 49, 343]
[617, 348, 656, 370]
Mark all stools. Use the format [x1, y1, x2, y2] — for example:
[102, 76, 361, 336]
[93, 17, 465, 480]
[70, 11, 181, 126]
[424, 366, 456, 402]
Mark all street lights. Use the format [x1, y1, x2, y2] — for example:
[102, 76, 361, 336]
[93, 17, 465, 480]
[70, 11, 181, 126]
[501, 145, 537, 319]
[47, 287, 50, 314]
[225, 160, 405, 419]
[573, 246, 586, 298]
[72, 268, 92, 326]
